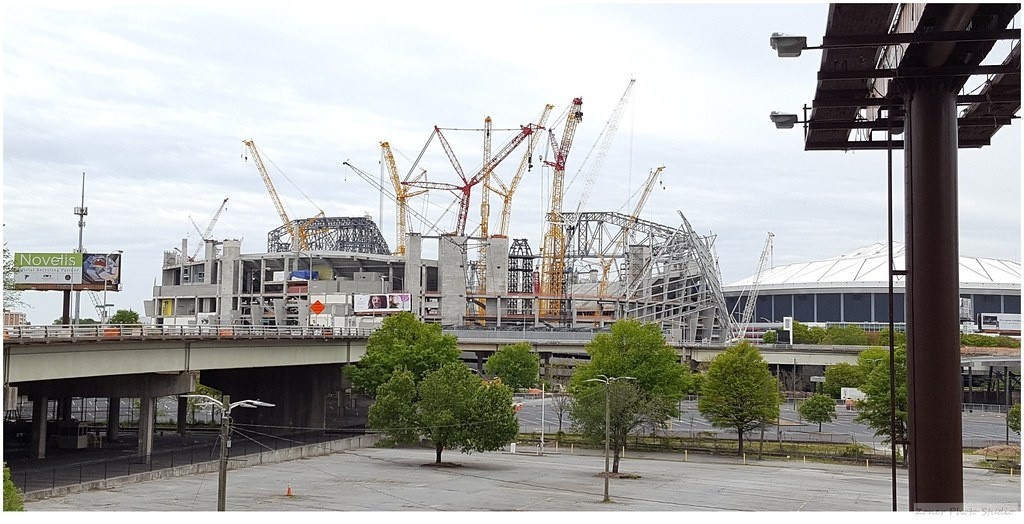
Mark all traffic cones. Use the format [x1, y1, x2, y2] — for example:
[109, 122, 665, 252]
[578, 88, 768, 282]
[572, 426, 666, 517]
[285, 482, 294, 496]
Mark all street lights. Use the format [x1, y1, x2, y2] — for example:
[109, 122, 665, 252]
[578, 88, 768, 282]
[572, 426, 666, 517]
[180, 394, 276, 511]
[585, 374, 638, 503]
[103, 250, 124, 323]
[292, 250, 312, 327]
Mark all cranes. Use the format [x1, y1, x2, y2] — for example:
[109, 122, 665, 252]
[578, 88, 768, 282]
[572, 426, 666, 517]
[177, 77, 776, 345]
